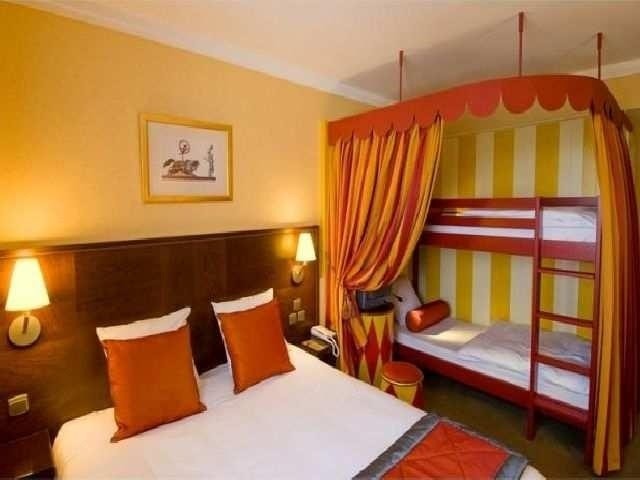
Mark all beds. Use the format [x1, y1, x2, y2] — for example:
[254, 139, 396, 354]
[393, 197, 600, 267]
[393, 313, 594, 432]
[54, 341, 547, 480]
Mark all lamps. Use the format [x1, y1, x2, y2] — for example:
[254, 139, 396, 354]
[289, 233, 318, 285]
[5, 256, 52, 348]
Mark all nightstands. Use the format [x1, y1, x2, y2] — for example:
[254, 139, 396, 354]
[302, 337, 340, 366]
[0, 429, 55, 480]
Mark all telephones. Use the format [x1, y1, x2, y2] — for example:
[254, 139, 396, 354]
[309, 325, 340, 357]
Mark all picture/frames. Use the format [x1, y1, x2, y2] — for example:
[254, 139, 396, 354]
[138, 112, 235, 206]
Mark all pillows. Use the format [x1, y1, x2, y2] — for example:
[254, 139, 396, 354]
[95, 307, 197, 389]
[406, 300, 450, 332]
[103, 326, 207, 443]
[394, 279, 423, 327]
[210, 289, 275, 389]
[217, 302, 296, 395]
